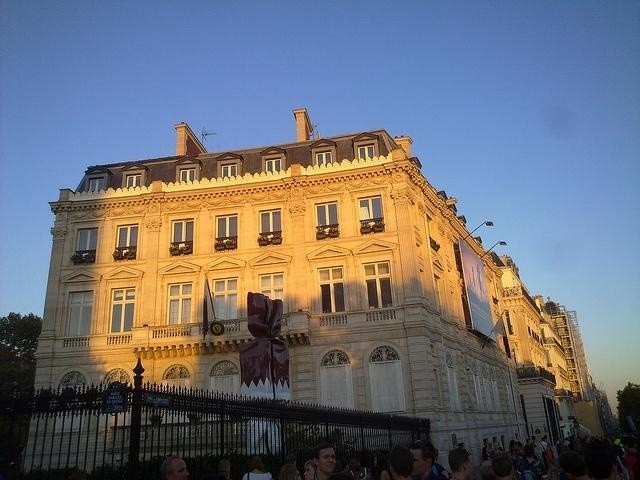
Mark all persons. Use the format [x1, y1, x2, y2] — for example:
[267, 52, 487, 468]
[304, 461, 318, 478]
[279, 456, 300, 478]
[161, 456, 189, 479]
[313, 443, 353, 478]
[217, 455, 231, 478]
[243, 456, 272, 478]
[337, 430, 640, 479]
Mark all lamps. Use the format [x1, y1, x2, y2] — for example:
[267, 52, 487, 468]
[463, 220, 507, 259]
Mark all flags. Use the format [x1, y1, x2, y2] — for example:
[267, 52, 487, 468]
[202, 282, 213, 338]
